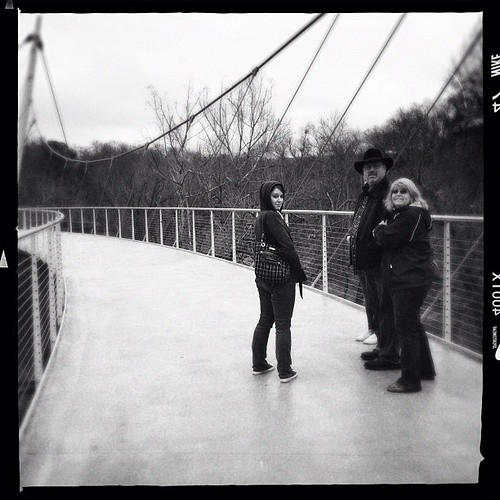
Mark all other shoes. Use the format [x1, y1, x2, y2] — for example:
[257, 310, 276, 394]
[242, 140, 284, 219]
[421, 372, 435, 380]
[363, 333, 377, 344]
[364, 357, 400, 370]
[387, 378, 421, 393]
[356, 331, 372, 341]
[360, 349, 379, 360]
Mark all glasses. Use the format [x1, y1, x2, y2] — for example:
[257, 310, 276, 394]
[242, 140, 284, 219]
[363, 163, 385, 169]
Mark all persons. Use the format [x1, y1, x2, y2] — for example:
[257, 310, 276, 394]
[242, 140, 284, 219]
[372, 178, 436, 392]
[251, 180, 311, 383]
[346, 149, 401, 371]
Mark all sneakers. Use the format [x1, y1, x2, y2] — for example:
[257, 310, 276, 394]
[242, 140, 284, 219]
[279, 370, 298, 382]
[252, 364, 274, 375]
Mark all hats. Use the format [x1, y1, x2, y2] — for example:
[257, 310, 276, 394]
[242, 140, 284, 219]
[354, 149, 393, 174]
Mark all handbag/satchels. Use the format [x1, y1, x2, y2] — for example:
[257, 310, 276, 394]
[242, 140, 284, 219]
[252, 209, 291, 284]
